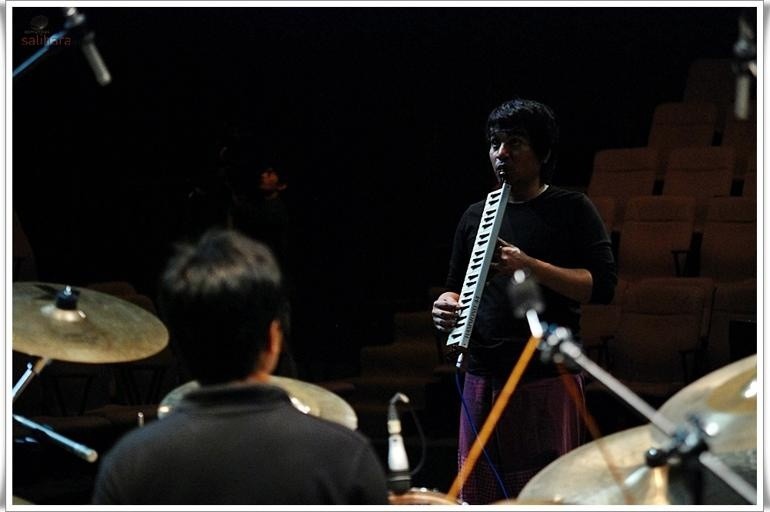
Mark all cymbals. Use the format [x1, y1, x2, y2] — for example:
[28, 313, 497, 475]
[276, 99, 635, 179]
[157, 373, 359, 432]
[648, 353, 757, 452]
[518, 424, 758, 506]
[13, 282, 170, 363]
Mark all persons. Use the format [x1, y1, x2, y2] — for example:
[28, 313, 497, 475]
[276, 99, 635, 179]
[432, 101, 616, 505]
[260, 163, 287, 198]
[92, 233, 388, 506]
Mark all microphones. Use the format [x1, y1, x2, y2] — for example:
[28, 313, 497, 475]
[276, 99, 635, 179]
[386, 393, 412, 496]
[59, 7, 114, 88]
[507, 268, 546, 338]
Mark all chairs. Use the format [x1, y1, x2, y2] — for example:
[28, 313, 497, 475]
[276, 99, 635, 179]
[697, 195, 758, 323]
[723, 120, 759, 148]
[596, 279, 715, 394]
[657, 148, 737, 208]
[611, 197, 697, 305]
[683, 60, 747, 132]
[646, 103, 719, 153]
[587, 150, 653, 237]
[742, 147, 759, 193]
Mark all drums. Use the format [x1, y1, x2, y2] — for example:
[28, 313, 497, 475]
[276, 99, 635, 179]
[387, 487, 468, 505]
